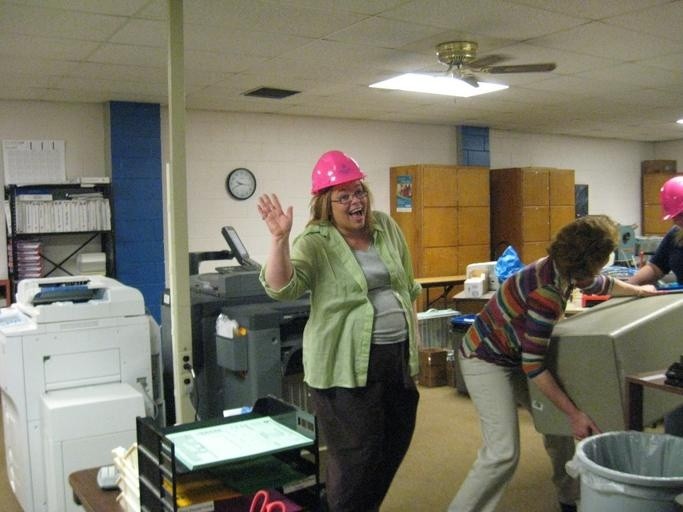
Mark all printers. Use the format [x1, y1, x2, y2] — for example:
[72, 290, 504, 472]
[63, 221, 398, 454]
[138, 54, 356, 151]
[190, 266, 312, 419]
[1, 274, 166, 512]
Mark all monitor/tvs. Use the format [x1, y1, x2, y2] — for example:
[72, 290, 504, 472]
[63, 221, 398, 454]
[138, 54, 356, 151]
[221, 226, 249, 264]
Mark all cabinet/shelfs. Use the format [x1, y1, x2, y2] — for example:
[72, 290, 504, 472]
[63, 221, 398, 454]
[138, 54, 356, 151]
[134, 396, 320, 511]
[388, 165, 576, 314]
[4, 182, 114, 304]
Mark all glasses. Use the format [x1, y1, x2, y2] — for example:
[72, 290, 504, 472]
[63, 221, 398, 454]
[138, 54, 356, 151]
[330, 190, 368, 204]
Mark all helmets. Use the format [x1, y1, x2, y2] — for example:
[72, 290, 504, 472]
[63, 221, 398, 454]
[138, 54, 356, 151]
[659, 176, 683, 221]
[311, 151, 363, 193]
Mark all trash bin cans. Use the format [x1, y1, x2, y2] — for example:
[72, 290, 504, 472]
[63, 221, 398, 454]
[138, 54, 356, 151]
[565, 430, 682, 512]
[451, 313, 477, 395]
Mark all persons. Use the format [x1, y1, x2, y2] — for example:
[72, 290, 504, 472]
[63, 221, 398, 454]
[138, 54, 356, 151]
[624, 175, 682, 288]
[253, 147, 423, 511]
[445, 212, 671, 511]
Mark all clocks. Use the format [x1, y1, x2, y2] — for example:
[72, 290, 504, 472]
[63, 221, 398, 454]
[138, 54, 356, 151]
[226, 168, 256, 201]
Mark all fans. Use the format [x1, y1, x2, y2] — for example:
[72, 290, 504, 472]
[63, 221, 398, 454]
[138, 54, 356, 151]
[411, 42, 557, 88]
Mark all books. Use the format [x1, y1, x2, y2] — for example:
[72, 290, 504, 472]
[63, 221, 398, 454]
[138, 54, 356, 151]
[0, 175, 109, 302]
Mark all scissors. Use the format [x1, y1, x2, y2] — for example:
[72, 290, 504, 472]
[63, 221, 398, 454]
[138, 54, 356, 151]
[246, 488, 286, 512]
